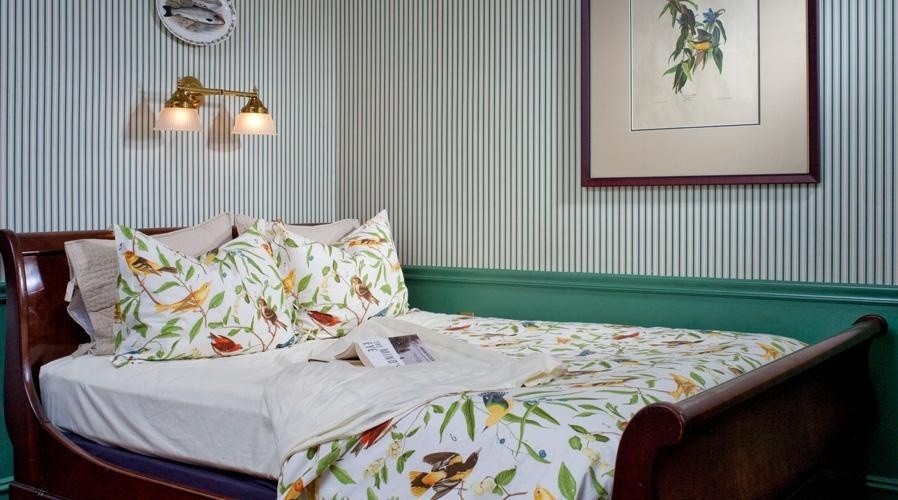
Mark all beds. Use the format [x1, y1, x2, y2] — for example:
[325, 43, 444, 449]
[0, 223, 898, 500]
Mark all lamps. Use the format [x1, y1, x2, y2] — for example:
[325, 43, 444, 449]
[152, 76, 277, 135]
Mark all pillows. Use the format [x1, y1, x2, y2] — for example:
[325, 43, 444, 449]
[66, 212, 235, 357]
[112, 223, 309, 366]
[233, 213, 360, 245]
[269, 222, 408, 341]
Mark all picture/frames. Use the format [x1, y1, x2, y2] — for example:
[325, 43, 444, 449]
[581, 0, 820, 187]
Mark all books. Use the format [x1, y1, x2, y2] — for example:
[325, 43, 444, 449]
[334, 332, 436, 371]
